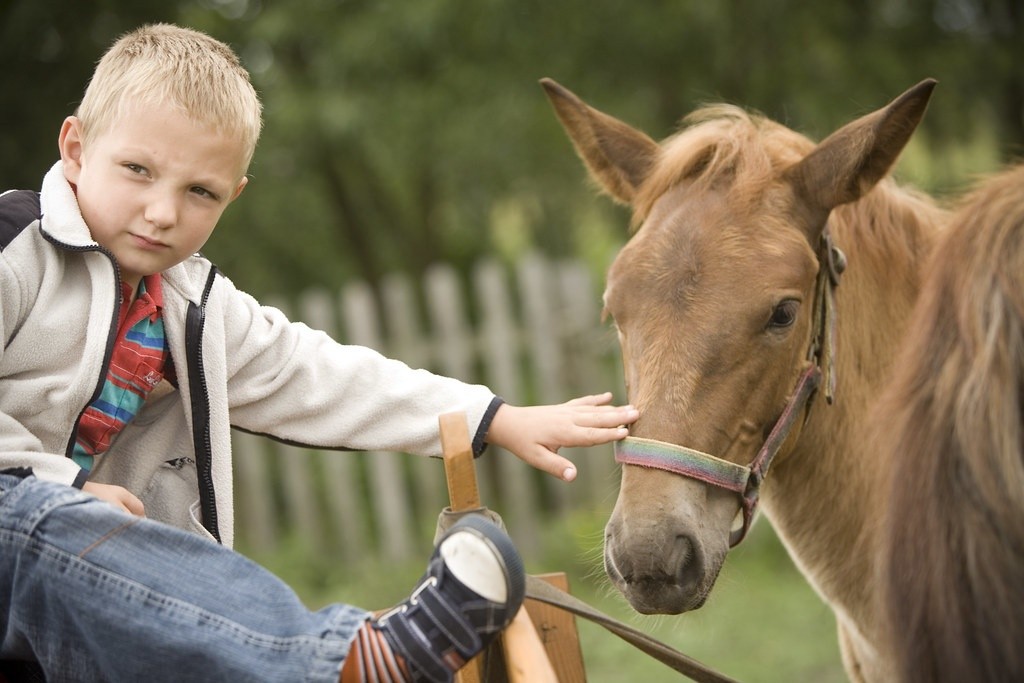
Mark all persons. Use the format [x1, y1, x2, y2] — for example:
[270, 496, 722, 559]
[0, 23, 640, 683]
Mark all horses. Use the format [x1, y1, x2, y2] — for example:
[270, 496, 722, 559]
[534, 76, 1024, 683]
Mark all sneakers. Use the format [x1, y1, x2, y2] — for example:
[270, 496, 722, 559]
[371, 513, 526, 683]
